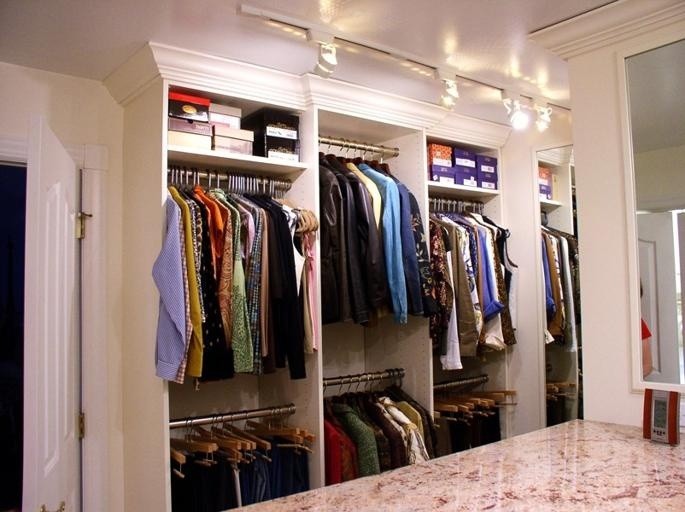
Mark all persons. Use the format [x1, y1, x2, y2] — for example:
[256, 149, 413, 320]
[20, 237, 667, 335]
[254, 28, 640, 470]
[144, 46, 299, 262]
[640, 279, 652, 380]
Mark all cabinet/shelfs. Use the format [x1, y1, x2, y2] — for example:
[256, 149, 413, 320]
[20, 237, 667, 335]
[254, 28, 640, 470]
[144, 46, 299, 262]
[535, 163, 580, 425]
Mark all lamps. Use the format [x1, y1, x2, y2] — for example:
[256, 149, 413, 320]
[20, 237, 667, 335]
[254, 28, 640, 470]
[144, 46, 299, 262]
[312, 43, 338, 79]
[502, 97, 554, 132]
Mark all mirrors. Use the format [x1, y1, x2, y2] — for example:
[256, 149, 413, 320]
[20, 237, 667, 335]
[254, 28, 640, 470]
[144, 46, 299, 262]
[533, 143, 584, 427]
[623, 37, 685, 388]
